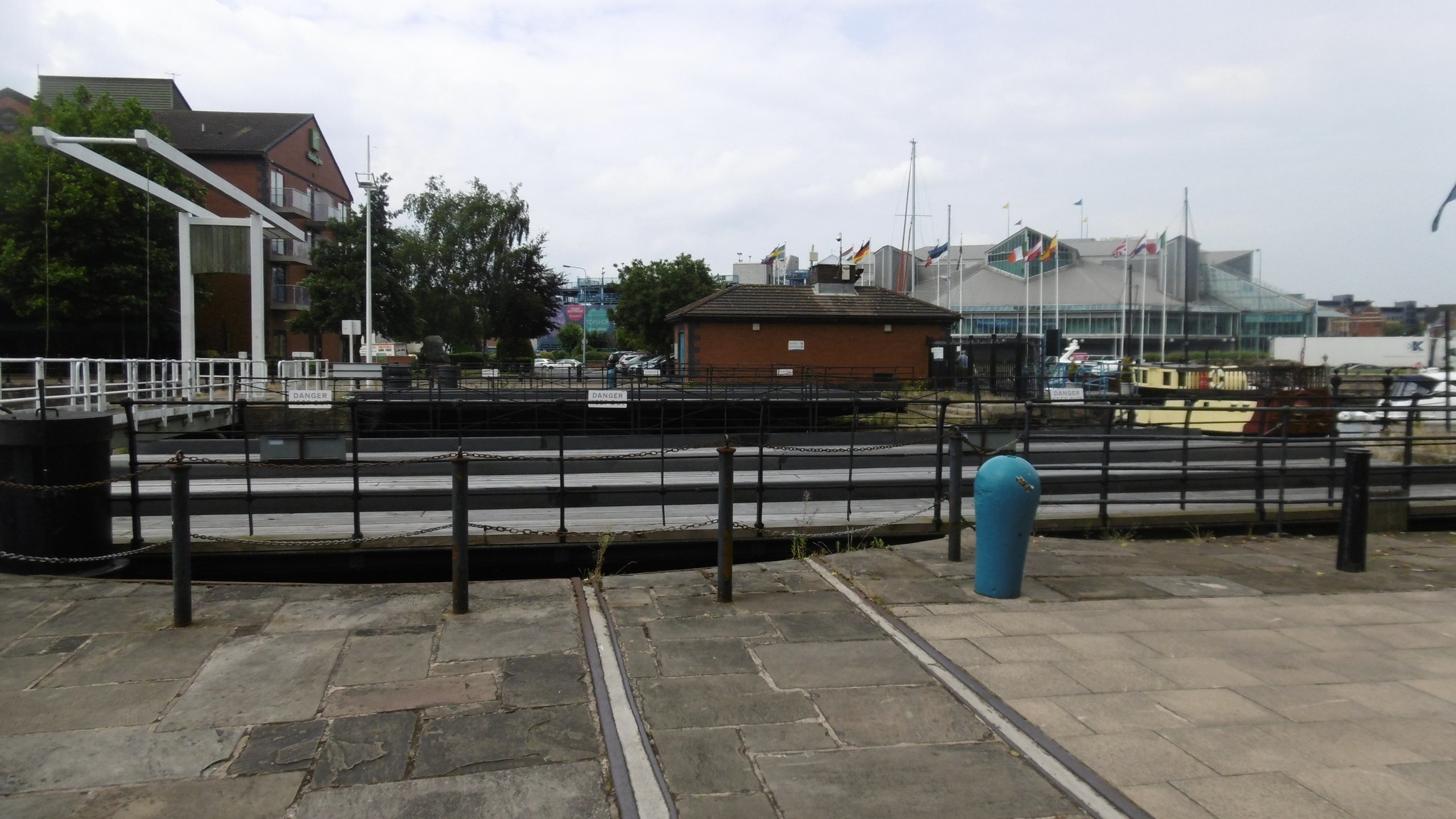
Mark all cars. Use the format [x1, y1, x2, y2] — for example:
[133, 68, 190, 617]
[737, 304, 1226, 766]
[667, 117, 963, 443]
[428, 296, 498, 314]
[532, 357, 586, 371]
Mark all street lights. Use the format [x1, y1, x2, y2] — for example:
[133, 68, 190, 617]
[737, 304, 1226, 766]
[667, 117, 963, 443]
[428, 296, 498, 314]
[563, 265, 587, 369]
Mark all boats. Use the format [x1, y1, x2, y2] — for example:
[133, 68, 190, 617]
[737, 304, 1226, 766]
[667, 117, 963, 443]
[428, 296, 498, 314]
[1336, 368, 1456, 450]
[1112, 363, 1338, 441]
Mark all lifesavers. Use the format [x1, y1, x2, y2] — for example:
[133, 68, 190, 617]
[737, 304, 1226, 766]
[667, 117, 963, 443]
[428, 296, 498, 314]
[1283, 391, 1322, 432]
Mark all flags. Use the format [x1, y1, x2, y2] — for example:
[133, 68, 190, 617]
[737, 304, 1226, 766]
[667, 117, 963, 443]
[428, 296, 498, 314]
[1432, 184, 1456, 231]
[762, 239, 871, 263]
[926, 233, 1058, 267]
[1112, 232, 1166, 257]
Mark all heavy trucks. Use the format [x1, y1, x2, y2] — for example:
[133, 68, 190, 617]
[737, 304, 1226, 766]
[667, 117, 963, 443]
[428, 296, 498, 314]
[1269, 336, 1455, 371]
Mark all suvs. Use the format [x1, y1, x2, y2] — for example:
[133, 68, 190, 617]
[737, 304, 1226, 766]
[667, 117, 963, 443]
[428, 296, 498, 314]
[607, 349, 675, 376]
